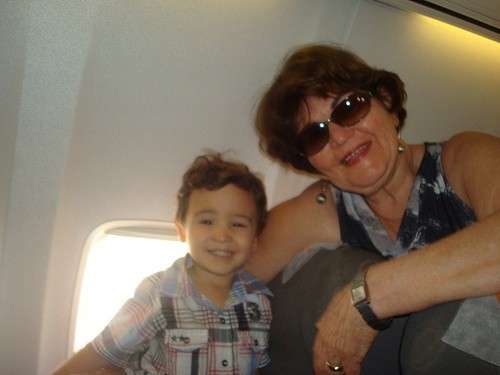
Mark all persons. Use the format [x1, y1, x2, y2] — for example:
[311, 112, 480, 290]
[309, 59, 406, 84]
[241, 45, 499, 375]
[54, 148, 273, 375]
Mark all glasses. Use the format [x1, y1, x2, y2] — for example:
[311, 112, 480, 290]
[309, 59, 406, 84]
[294, 90, 377, 160]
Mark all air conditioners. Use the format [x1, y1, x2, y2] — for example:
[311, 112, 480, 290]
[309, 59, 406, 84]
[373, 0, 500, 42]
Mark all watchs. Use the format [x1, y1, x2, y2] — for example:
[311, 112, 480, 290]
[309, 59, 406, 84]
[350, 267, 393, 331]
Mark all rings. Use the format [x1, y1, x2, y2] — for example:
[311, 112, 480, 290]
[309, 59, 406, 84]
[327, 362, 342, 373]
